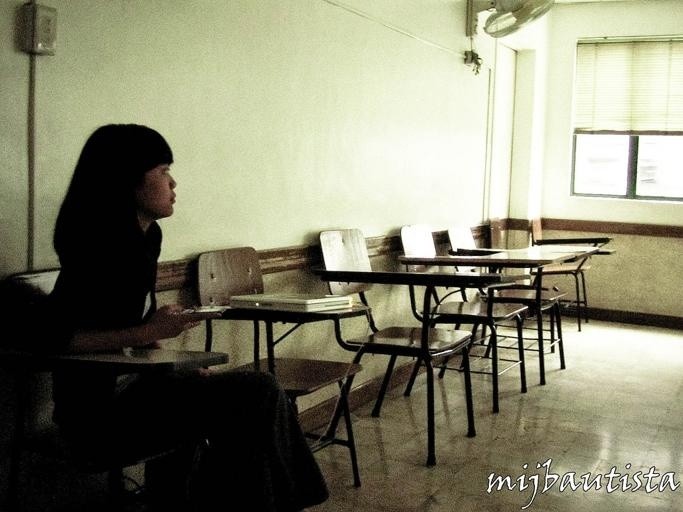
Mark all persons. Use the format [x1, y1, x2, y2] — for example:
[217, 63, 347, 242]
[45, 123, 329, 512]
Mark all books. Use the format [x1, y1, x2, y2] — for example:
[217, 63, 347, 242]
[231, 293, 354, 311]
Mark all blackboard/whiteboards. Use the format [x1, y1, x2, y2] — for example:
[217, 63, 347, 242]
[28, 0, 493, 275]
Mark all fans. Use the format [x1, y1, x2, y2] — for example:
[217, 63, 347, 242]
[465, 0, 557, 74]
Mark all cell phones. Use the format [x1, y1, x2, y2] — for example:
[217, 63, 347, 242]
[172, 304, 228, 316]
